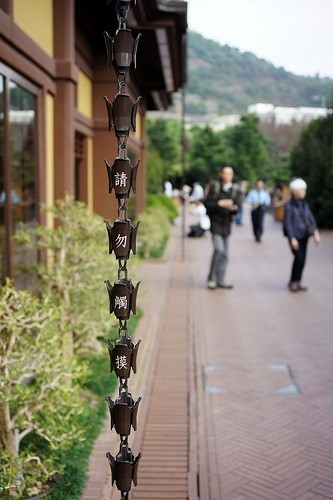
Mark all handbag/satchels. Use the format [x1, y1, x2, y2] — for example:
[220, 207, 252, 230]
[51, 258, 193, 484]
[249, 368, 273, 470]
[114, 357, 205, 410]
[306, 220, 313, 235]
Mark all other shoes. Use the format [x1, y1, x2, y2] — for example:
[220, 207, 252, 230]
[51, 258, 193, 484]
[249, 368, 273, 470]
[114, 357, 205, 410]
[290, 282, 297, 291]
[218, 282, 232, 288]
[297, 284, 307, 290]
[208, 280, 217, 289]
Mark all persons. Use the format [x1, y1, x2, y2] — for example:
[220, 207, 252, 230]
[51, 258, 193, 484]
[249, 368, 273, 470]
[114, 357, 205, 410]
[160, 180, 248, 238]
[202, 165, 242, 289]
[246, 179, 271, 243]
[283, 179, 321, 292]
